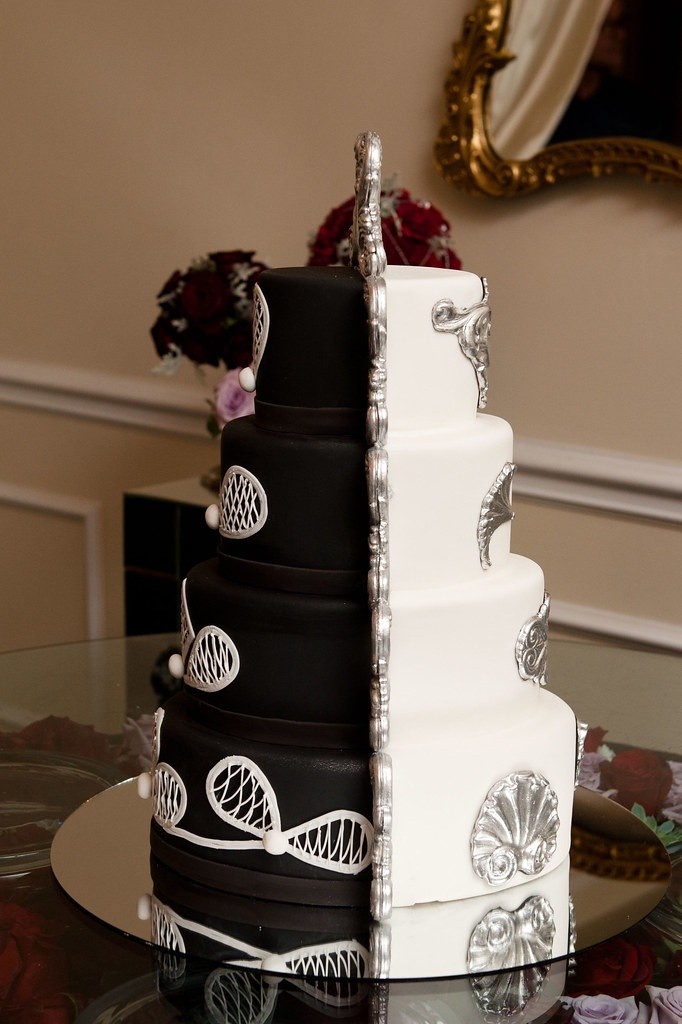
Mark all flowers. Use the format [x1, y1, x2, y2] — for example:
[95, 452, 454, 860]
[150, 187, 462, 436]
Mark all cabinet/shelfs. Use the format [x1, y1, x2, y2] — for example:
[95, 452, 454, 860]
[121, 482, 220, 635]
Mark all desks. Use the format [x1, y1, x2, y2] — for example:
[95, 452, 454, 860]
[0, 630, 682, 1024]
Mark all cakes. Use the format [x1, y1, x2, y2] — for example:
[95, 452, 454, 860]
[135, 131, 579, 921]
[138, 855, 578, 1024]
